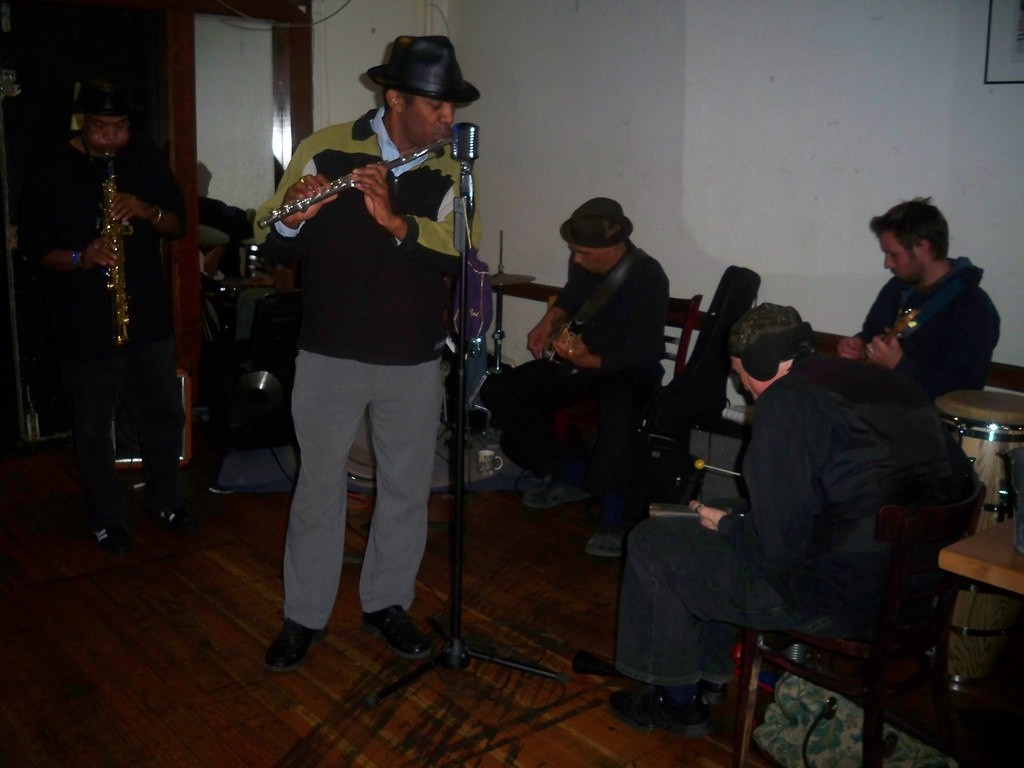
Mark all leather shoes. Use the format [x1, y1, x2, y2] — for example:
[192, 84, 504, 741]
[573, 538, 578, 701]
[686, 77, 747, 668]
[146, 502, 199, 537]
[609, 680, 712, 741]
[263, 618, 329, 673]
[88, 509, 132, 556]
[361, 603, 432, 661]
[699, 678, 728, 707]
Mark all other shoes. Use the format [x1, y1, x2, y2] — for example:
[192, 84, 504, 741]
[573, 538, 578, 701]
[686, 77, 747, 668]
[585, 526, 630, 557]
[516, 479, 588, 510]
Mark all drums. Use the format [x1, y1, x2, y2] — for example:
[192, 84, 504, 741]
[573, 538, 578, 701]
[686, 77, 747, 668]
[932, 390, 1024, 693]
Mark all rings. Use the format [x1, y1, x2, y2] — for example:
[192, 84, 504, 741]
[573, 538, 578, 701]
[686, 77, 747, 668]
[868, 348, 875, 353]
[695, 504, 705, 513]
[568, 350, 574, 353]
[298, 176, 306, 183]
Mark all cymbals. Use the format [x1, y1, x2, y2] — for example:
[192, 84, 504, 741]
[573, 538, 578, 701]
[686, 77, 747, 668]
[489, 272, 536, 287]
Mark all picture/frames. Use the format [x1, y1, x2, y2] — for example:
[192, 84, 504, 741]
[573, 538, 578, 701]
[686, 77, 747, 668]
[984, 0, 1024, 84]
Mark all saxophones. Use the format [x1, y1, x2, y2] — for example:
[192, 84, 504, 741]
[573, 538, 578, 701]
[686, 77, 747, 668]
[100, 146, 134, 349]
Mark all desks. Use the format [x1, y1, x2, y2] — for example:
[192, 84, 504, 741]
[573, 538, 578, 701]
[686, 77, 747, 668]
[936, 515, 1024, 597]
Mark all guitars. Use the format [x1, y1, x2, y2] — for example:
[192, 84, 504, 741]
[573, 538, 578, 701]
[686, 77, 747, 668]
[537, 317, 586, 401]
[858, 308, 921, 368]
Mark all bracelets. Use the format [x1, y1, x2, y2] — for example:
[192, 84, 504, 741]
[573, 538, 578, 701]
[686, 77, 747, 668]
[211, 250, 222, 259]
[71, 250, 82, 269]
[149, 205, 166, 228]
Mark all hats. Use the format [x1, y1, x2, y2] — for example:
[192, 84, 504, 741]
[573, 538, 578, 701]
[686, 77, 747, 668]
[367, 35, 480, 102]
[66, 69, 133, 116]
[727, 301, 808, 362]
[559, 197, 633, 249]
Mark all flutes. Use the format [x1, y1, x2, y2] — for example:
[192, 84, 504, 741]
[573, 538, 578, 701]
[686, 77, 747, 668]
[255, 135, 453, 228]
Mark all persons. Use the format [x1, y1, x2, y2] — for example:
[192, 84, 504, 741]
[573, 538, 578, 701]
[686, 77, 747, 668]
[255, 37, 483, 671]
[19, 87, 200, 553]
[196, 161, 255, 343]
[839, 196, 999, 405]
[489, 199, 669, 557]
[611, 303, 978, 740]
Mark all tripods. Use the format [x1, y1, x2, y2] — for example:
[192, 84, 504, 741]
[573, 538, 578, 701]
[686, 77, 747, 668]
[361, 163, 569, 713]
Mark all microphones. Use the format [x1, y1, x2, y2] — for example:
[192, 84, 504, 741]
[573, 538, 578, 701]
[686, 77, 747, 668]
[449, 122, 479, 160]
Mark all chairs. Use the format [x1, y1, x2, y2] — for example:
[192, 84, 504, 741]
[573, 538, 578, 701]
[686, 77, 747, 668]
[554, 293, 703, 462]
[732, 478, 988, 768]
